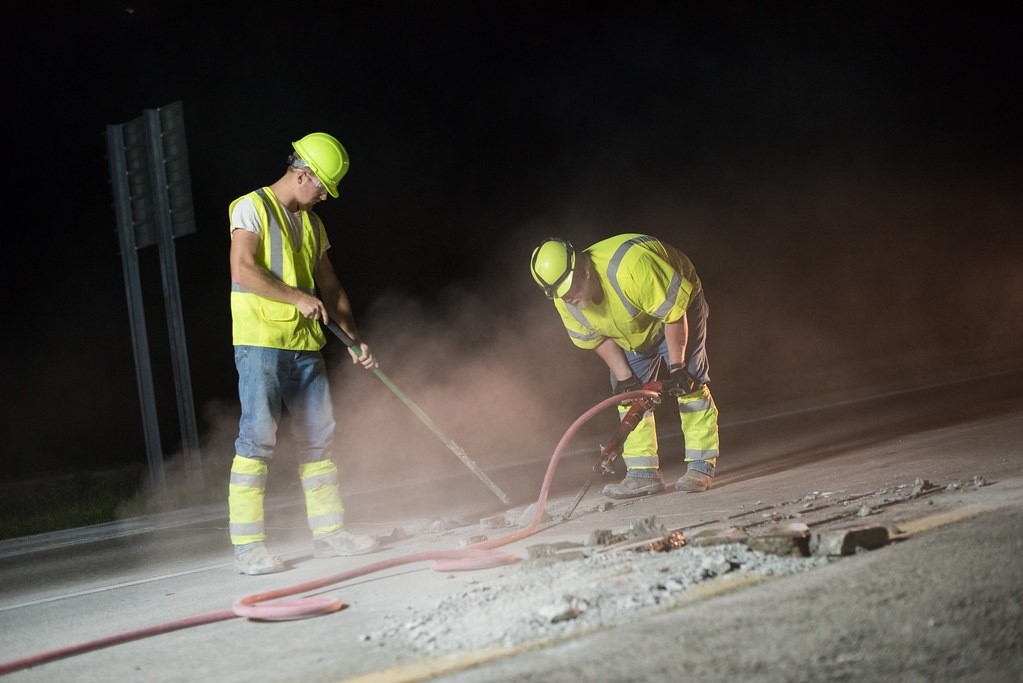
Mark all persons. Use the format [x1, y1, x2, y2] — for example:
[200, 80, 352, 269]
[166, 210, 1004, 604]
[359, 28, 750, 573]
[530, 233, 720, 500]
[226, 133, 380, 575]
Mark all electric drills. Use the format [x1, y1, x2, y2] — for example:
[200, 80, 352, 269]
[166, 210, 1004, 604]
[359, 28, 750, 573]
[555, 354, 699, 519]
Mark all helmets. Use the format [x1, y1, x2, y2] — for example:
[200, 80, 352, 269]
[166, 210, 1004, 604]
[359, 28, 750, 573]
[292, 132, 350, 198]
[529, 236, 575, 299]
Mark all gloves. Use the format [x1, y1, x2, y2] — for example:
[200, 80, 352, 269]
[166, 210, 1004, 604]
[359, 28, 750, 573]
[669, 362, 694, 397]
[618, 376, 644, 393]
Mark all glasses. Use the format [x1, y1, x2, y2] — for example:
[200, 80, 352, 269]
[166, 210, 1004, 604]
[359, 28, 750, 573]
[304, 172, 327, 194]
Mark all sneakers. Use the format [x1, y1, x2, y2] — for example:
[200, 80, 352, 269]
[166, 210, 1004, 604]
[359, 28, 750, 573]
[314, 531, 376, 559]
[234, 545, 285, 575]
[603, 476, 665, 499]
[677, 468, 712, 492]
